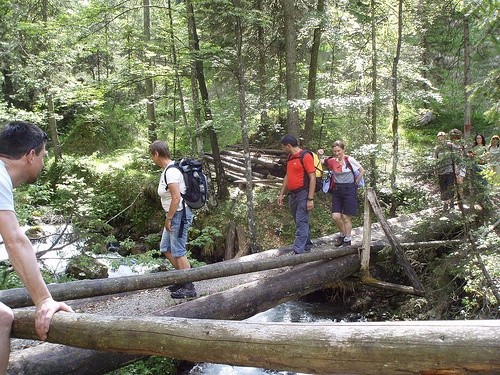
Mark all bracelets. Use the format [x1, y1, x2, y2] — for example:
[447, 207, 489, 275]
[308, 198, 313, 201]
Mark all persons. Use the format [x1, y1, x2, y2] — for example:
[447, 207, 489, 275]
[487, 135, 500, 193]
[148, 140, 196, 299]
[433, 132, 463, 212]
[0, 120, 75, 375]
[470, 134, 488, 186]
[317, 141, 365, 247]
[447, 129, 468, 211]
[277, 135, 316, 255]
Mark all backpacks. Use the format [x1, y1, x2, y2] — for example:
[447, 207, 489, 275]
[286, 150, 323, 192]
[164, 156, 208, 209]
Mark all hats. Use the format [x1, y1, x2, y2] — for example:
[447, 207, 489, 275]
[436, 132, 446, 137]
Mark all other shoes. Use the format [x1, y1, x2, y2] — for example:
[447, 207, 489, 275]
[334, 235, 346, 246]
[303, 249, 310, 253]
[279, 250, 295, 257]
[171, 288, 196, 297]
[168, 283, 182, 292]
[339, 238, 351, 248]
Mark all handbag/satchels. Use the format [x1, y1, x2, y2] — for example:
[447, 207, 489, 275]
[355, 171, 364, 187]
[329, 174, 335, 192]
[323, 170, 332, 193]
